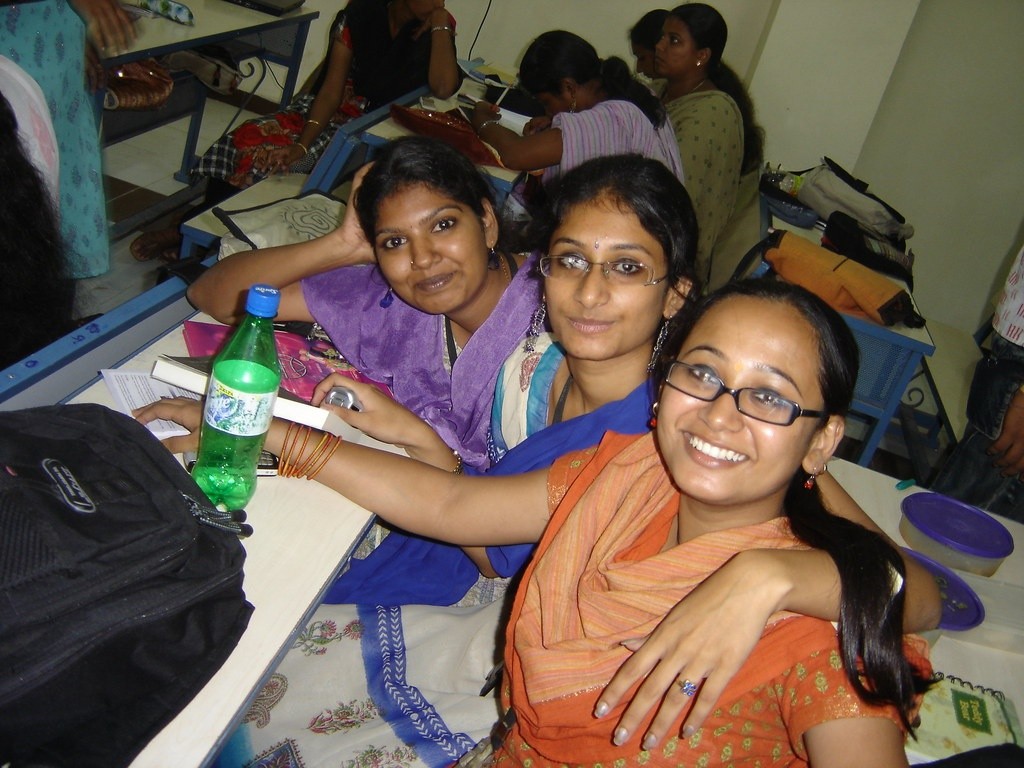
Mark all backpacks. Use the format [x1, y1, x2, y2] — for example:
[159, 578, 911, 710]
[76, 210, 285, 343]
[0, 402, 254, 768]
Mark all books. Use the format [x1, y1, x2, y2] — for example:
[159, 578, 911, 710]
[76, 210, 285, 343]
[458, 105, 473, 124]
[152, 354, 330, 430]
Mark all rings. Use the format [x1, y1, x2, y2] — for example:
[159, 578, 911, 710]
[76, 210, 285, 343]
[676, 677, 698, 696]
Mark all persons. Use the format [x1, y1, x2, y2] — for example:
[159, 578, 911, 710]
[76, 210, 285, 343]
[210, 151, 942, 768]
[0, 1, 137, 371]
[475, 30, 684, 235]
[129, 0, 464, 261]
[657, 3, 764, 288]
[630, 9, 670, 79]
[186, 134, 542, 469]
[133, 280, 947, 768]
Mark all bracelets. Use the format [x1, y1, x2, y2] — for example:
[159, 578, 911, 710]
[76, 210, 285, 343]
[431, 26, 452, 33]
[297, 143, 307, 154]
[278, 421, 343, 480]
[453, 454, 462, 473]
[477, 121, 499, 138]
[305, 119, 325, 129]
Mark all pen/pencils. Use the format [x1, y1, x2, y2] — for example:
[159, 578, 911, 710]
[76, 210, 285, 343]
[494, 85, 510, 106]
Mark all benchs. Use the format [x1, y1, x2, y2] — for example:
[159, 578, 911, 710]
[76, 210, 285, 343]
[898, 317, 983, 486]
[706, 166, 780, 299]
[180, 84, 431, 259]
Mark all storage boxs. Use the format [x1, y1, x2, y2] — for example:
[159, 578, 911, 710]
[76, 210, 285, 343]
[940, 568, 1024, 655]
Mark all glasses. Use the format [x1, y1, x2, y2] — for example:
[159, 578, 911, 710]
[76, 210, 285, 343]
[541, 251, 671, 289]
[660, 354, 830, 427]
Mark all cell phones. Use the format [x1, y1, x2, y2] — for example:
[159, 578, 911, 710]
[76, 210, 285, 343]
[324, 387, 365, 414]
[183, 448, 278, 477]
[419, 97, 436, 110]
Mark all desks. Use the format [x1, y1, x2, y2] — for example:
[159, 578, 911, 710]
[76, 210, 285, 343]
[827, 454, 1024, 768]
[102, 0, 320, 186]
[769, 215, 937, 468]
[0, 308, 408, 768]
[360, 78, 525, 203]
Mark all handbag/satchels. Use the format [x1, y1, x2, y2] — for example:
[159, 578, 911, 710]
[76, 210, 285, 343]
[798, 161, 914, 241]
[827, 208, 914, 296]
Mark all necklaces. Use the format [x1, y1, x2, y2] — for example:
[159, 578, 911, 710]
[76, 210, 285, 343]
[501, 256, 510, 281]
[688, 77, 708, 93]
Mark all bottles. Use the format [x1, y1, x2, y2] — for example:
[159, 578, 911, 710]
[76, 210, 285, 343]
[192, 286, 282, 509]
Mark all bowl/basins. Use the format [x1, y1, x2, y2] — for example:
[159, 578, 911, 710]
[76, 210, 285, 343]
[898, 492, 1014, 580]
